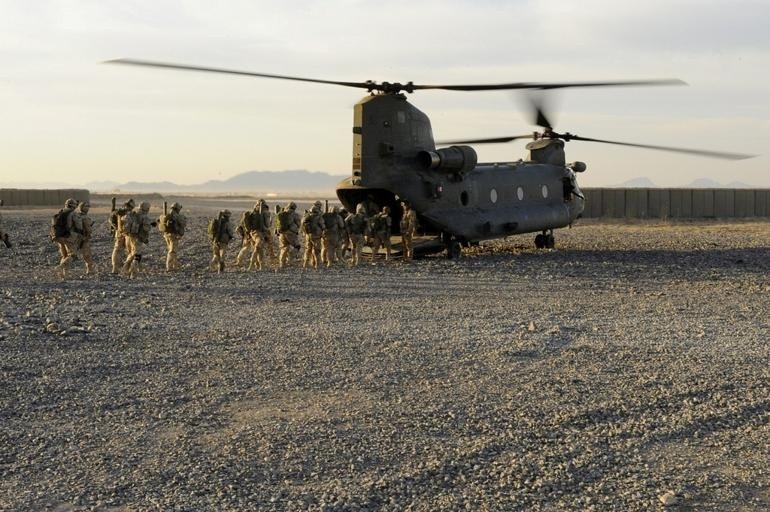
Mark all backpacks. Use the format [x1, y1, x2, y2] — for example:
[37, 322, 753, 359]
[275, 211, 289, 234]
[351, 214, 364, 235]
[157, 214, 176, 232]
[120, 210, 140, 234]
[243, 212, 262, 231]
[301, 213, 317, 234]
[323, 212, 337, 229]
[368, 215, 386, 233]
[208, 218, 225, 243]
[54, 208, 73, 238]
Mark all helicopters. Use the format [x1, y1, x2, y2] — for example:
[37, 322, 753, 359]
[103, 55, 762, 263]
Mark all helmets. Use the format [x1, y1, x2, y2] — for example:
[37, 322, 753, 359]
[330, 200, 410, 214]
[255, 198, 269, 211]
[64, 199, 77, 208]
[124, 199, 135, 208]
[78, 201, 90, 211]
[170, 202, 182, 210]
[219, 208, 232, 218]
[309, 201, 322, 213]
[139, 200, 151, 209]
[286, 201, 297, 209]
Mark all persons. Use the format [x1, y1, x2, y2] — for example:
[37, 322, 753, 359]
[49, 197, 77, 280]
[110, 197, 136, 274]
[116, 200, 151, 280]
[163, 201, 187, 272]
[72, 199, 98, 278]
[228, 198, 419, 274]
[206, 208, 235, 274]
